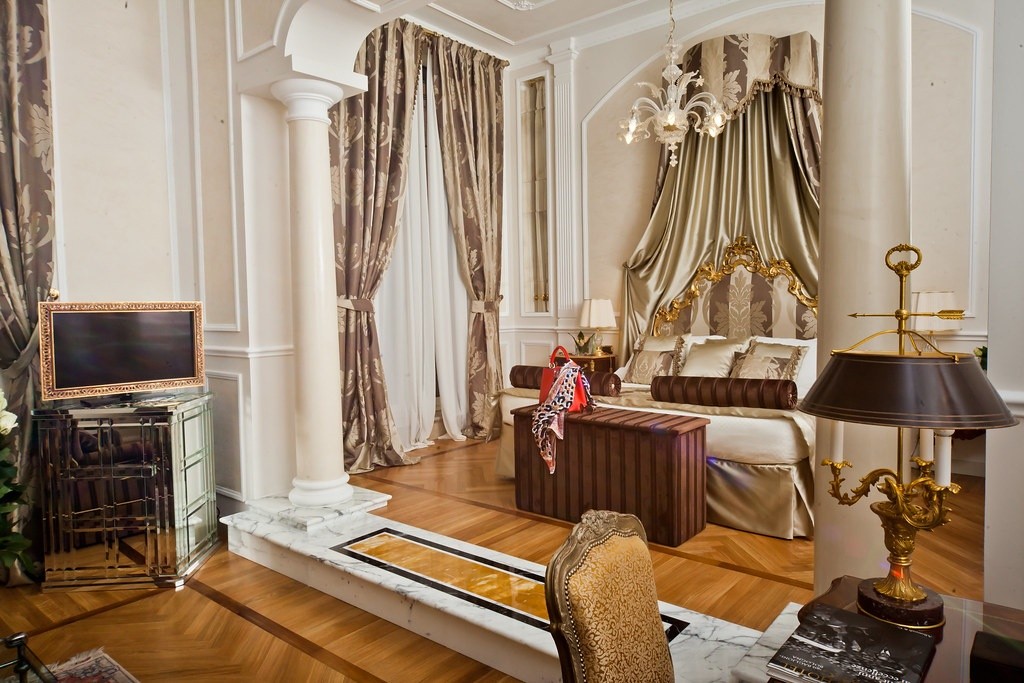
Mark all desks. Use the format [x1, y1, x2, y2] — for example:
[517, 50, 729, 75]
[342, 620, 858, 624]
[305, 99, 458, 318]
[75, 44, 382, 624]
[29, 391, 224, 593]
[0, 618, 59, 683]
[764, 575, 1024, 683]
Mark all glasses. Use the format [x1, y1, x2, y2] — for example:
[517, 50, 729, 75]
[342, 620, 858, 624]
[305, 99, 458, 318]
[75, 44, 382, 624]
[579, 403, 593, 415]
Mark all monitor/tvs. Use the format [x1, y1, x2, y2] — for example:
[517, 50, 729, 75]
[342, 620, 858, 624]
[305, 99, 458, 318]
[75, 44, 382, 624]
[36, 300, 206, 406]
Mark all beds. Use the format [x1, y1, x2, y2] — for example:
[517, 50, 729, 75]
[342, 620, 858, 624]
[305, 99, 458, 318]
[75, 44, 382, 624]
[484, 237, 815, 541]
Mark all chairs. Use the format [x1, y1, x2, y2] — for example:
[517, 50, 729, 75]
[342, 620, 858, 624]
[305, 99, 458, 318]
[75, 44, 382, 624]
[544, 510, 675, 683]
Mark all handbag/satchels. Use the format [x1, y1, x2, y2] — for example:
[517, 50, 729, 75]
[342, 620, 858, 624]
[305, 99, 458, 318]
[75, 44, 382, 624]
[539, 346, 587, 413]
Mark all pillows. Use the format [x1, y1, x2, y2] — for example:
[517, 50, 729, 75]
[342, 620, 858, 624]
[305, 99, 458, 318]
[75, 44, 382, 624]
[748, 339, 809, 381]
[509, 364, 621, 397]
[635, 333, 691, 374]
[651, 376, 798, 409]
[729, 351, 791, 380]
[679, 342, 743, 378]
[706, 338, 748, 352]
[614, 335, 727, 381]
[622, 348, 677, 385]
[743, 336, 816, 399]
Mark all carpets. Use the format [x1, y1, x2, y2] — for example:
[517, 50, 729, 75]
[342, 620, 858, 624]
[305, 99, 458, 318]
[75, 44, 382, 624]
[0, 646, 142, 683]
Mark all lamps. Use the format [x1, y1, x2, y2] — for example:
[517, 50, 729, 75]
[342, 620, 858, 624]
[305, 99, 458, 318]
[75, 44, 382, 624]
[793, 242, 1021, 631]
[579, 298, 618, 356]
[910, 290, 963, 351]
[617, 0, 727, 167]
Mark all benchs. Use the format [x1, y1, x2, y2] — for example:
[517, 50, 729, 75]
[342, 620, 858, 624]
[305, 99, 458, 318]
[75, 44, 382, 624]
[510, 404, 711, 548]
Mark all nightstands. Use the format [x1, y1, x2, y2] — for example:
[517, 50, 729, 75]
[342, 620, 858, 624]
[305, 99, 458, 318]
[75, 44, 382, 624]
[549, 352, 616, 373]
